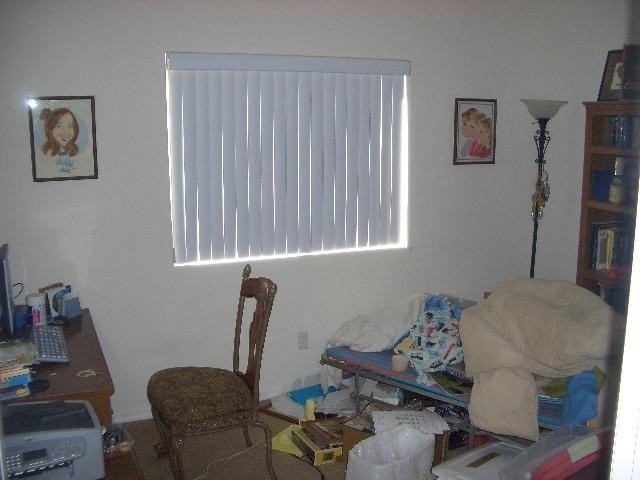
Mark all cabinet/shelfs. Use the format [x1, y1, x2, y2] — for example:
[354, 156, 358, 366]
[575, 100, 640, 315]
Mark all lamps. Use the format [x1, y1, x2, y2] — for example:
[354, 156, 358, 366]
[519, 97, 568, 280]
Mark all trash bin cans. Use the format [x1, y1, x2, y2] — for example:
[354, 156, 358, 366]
[102, 423, 146, 480]
[349, 425, 436, 480]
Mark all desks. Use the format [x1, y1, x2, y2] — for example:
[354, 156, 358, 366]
[98, 424, 144, 478]
[1, 304, 114, 438]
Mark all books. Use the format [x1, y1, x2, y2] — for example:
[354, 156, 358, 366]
[589, 219, 627, 314]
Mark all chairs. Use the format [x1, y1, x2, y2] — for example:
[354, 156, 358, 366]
[147, 264, 279, 480]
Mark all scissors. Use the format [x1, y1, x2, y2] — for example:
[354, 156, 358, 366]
[0, 379, 51, 401]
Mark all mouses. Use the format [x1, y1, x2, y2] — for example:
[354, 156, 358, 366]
[48, 315, 70, 325]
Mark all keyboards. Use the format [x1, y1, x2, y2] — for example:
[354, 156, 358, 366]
[32, 325, 70, 363]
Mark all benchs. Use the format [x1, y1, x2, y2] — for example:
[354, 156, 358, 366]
[322, 291, 609, 448]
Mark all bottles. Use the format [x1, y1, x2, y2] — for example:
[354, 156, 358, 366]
[605, 157, 625, 207]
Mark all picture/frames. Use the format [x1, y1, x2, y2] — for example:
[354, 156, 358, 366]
[27, 96, 100, 182]
[453, 97, 497, 166]
[597, 48, 627, 101]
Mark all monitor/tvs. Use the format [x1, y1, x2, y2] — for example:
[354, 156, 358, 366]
[0, 243, 24, 339]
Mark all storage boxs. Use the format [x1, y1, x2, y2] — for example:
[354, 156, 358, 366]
[273, 380, 613, 480]
[592, 170, 614, 205]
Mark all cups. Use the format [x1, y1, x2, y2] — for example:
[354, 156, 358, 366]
[391, 354, 408, 373]
[615, 116, 631, 147]
[13, 305, 29, 336]
